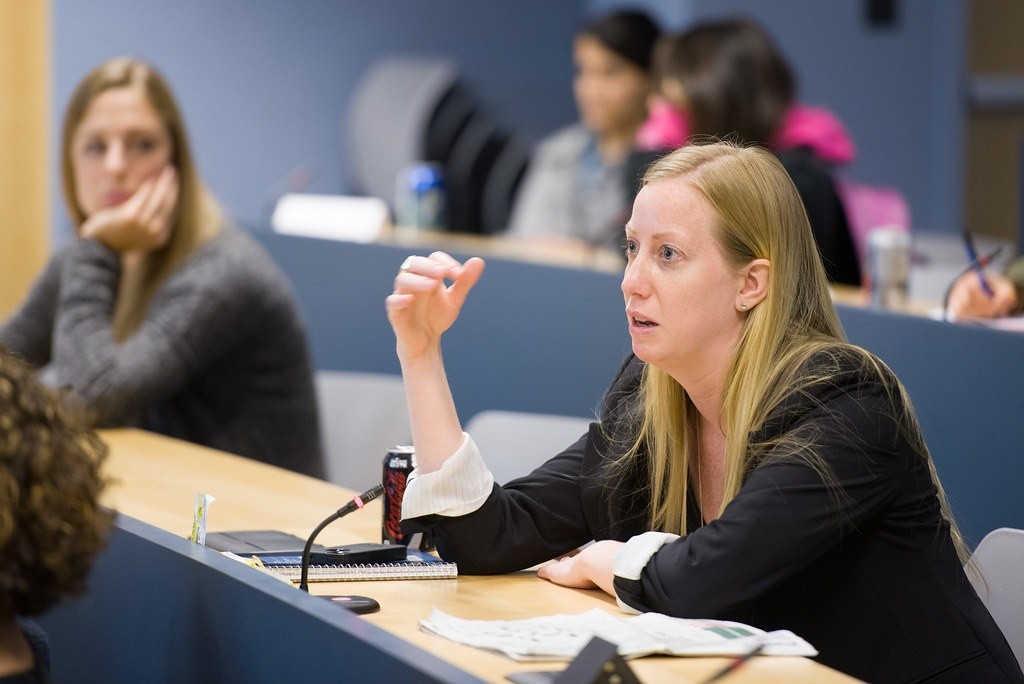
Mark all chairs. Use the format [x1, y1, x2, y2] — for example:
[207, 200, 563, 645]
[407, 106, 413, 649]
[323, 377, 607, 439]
[464, 409, 607, 486]
[964, 527, 1024, 684]
[314, 371, 415, 490]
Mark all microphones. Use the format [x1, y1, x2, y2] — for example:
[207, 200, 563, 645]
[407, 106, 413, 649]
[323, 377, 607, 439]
[943, 245, 1003, 322]
[299, 483, 386, 615]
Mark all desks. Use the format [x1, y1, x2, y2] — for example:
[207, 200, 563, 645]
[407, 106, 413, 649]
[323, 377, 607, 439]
[74, 427, 860, 684]
[278, 221, 1024, 546]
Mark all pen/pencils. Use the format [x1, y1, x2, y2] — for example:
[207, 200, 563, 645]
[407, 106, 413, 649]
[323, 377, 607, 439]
[960, 225, 994, 300]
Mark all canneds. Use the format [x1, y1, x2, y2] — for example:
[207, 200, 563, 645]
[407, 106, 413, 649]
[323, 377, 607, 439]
[396, 162, 449, 233]
[381, 446, 437, 549]
[864, 225, 911, 311]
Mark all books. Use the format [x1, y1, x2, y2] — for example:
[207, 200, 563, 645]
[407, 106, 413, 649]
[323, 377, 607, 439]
[235, 547, 458, 583]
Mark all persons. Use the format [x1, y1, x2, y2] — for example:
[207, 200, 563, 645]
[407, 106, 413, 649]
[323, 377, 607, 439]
[944, 256, 1024, 322]
[0, 350, 120, 684]
[384, 143, 1024, 684]
[502, 9, 865, 293]
[0, 57, 329, 482]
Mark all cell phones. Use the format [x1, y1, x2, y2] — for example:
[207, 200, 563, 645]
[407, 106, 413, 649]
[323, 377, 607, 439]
[308, 543, 407, 566]
